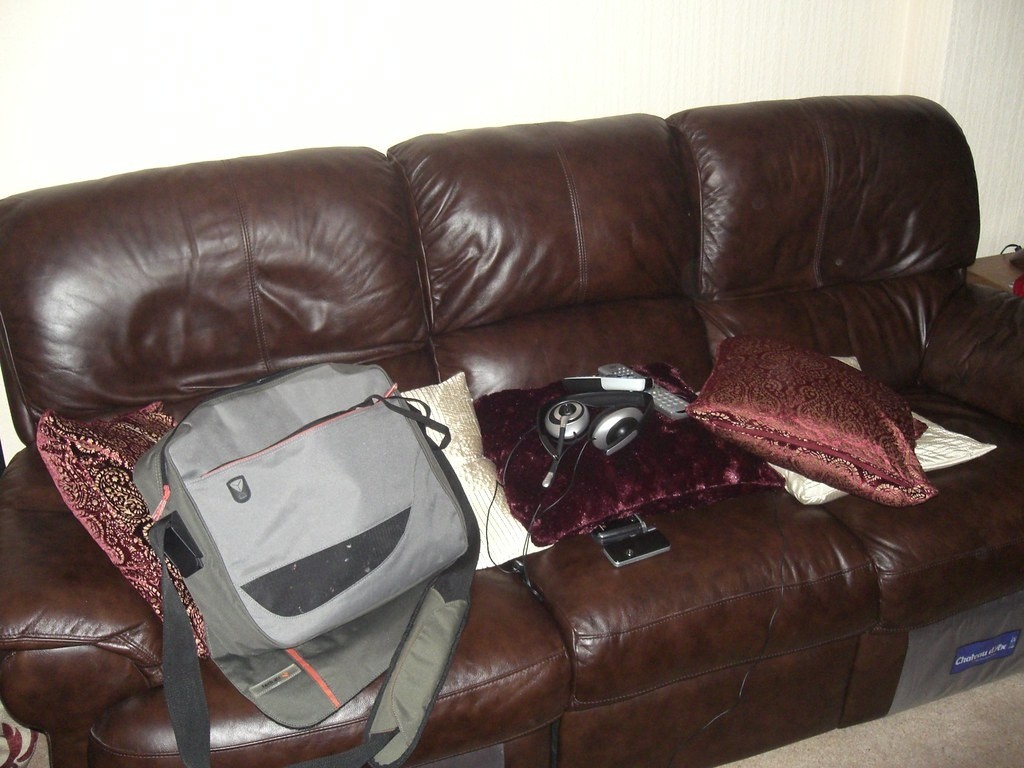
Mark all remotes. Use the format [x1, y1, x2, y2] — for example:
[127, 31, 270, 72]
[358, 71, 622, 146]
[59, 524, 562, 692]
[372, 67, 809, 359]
[598, 363, 690, 421]
[562, 377, 654, 394]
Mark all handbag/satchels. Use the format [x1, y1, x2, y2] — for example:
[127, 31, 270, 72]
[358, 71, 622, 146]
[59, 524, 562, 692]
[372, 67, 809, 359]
[133, 365, 481, 768]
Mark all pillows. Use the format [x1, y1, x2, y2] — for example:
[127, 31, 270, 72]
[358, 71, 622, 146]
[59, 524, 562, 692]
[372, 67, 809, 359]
[474, 385, 784, 546]
[689, 336, 938, 507]
[398, 373, 541, 570]
[37, 401, 210, 662]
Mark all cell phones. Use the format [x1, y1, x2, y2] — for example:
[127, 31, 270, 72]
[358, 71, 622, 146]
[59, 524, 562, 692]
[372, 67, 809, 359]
[590, 513, 646, 546]
[602, 527, 671, 568]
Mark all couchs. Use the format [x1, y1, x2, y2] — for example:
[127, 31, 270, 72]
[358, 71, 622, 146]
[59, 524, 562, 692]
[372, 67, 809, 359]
[0, 95, 1024, 768]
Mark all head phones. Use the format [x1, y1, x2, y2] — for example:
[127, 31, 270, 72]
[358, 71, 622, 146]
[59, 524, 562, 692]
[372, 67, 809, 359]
[536, 391, 657, 457]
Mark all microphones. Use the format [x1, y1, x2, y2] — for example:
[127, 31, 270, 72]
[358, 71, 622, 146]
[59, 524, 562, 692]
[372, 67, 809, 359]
[540, 414, 569, 489]
